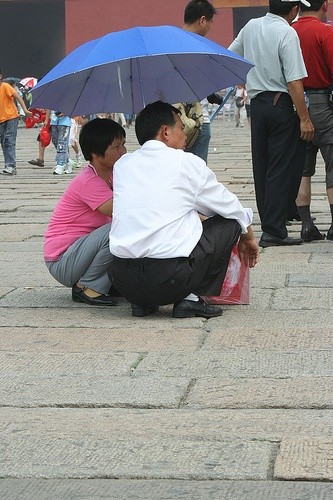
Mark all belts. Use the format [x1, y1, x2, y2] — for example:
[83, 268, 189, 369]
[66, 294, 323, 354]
[305, 89, 332, 94]
[273, 92, 282, 105]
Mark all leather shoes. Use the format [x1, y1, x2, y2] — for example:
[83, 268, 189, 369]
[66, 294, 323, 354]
[72, 284, 117, 306]
[173, 296, 223, 318]
[288, 212, 316, 222]
[131, 303, 160, 316]
[259, 232, 303, 246]
[301, 226, 326, 241]
[328, 226, 333, 239]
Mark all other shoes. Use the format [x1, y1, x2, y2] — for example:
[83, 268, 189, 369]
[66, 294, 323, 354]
[236, 122, 240, 127]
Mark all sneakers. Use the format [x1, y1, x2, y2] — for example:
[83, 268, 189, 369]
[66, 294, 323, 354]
[65, 164, 74, 174]
[3, 167, 16, 175]
[53, 167, 64, 174]
[77, 163, 83, 167]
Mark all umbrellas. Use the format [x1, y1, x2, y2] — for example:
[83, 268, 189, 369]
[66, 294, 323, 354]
[19, 76, 39, 88]
[3, 76, 21, 85]
[26, 26, 259, 116]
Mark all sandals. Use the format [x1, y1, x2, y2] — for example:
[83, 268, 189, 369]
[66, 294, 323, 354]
[28, 159, 44, 167]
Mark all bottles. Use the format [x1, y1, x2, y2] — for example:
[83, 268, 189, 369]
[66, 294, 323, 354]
[303, 92, 309, 109]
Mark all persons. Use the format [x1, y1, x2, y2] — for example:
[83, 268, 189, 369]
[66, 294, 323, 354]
[11, 82, 34, 115]
[287, 0, 333, 241]
[179, 0, 216, 165]
[51, 109, 75, 174]
[28, 108, 53, 167]
[43, 117, 129, 307]
[90, 112, 131, 126]
[232, 83, 247, 127]
[112, 100, 258, 319]
[68, 117, 86, 168]
[223, 0, 315, 246]
[0, 70, 34, 175]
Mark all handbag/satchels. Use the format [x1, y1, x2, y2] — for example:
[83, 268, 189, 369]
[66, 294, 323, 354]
[236, 97, 245, 107]
[172, 102, 201, 150]
[205, 239, 250, 305]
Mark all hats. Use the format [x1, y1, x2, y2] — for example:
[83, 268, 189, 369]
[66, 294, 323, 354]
[283, 0, 311, 8]
